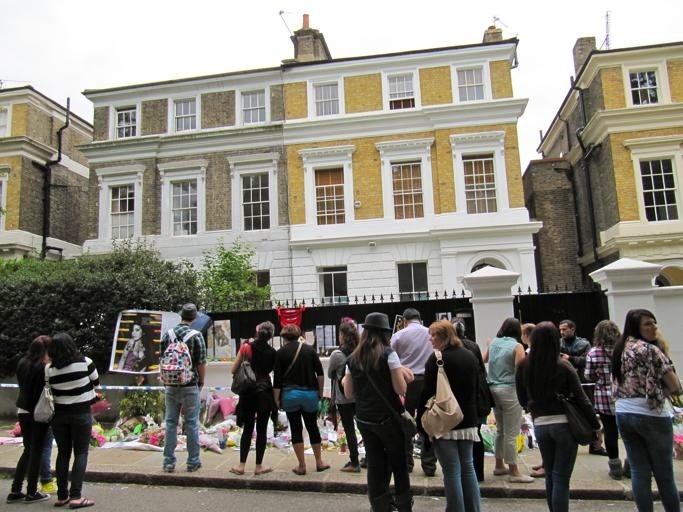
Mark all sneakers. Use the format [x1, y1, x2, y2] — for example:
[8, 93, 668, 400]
[25, 492, 51, 502]
[40, 482, 58, 494]
[162, 464, 175, 472]
[187, 462, 200, 472]
[7, 493, 26, 503]
[508, 473, 535, 484]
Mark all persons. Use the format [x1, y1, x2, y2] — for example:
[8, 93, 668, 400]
[272, 323, 330, 475]
[44, 333, 100, 508]
[118, 316, 155, 372]
[160, 303, 207, 473]
[40, 426, 58, 494]
[229, 320, 276, 475]
[6, 335, 51, 503]
[481, 308, 683, 512]
[328, 308, 493, 512]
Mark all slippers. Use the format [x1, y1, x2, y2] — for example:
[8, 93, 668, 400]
[54, 497, 94, 509]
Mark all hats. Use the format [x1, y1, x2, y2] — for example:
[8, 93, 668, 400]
[362, 312, 391, 332]
[181, 303, 197, 320]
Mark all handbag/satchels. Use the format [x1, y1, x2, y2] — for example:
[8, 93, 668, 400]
[420, 370, 464, 437]
[376, 413, 417, 474]
[231, 359, 257, 394]
[561, 394, 597, 446]
[34, 386, 55, 424]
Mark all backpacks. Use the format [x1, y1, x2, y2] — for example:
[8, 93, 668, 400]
[160, 329, 199, 385]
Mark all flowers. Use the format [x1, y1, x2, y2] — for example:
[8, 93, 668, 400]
[11, 388, 349, 460]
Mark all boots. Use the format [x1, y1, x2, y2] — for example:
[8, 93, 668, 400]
[609, 459, 622, 480]
